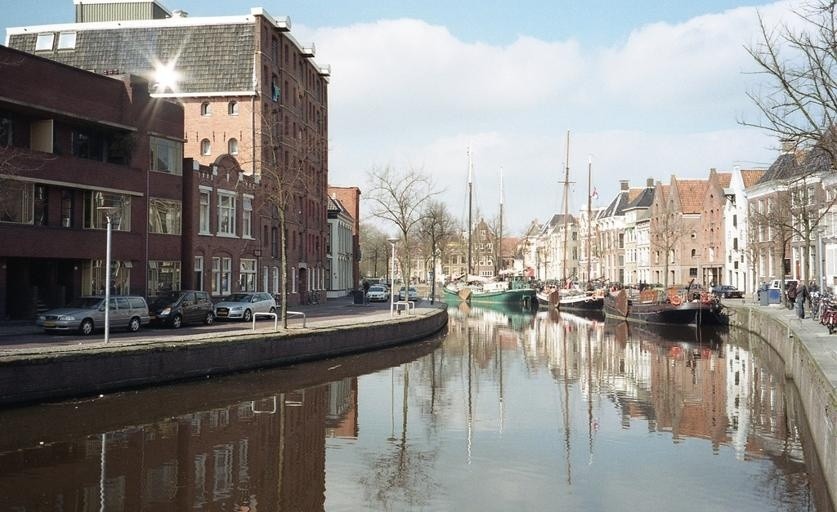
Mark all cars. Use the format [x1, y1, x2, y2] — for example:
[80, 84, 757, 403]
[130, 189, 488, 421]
[149, 290, 215, 329]
[366, 283, 390, 302]
[213, 291, 277, 322]
[712, 285, 743, 299]
[35, 295, 150, 336]
[399, 285, 417, 302]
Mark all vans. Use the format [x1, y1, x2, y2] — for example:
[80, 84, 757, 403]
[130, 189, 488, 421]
[768, 279, 801, 296]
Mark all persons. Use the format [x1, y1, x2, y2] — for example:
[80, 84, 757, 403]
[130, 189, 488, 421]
[794, 277, 805, 321]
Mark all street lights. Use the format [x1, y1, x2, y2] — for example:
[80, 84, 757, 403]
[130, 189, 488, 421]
[97, 206, 121, 344]
[387, 237, 401, 317]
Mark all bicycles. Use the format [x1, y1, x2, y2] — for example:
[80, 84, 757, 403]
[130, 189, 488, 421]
[809, 291, 837, 335]
